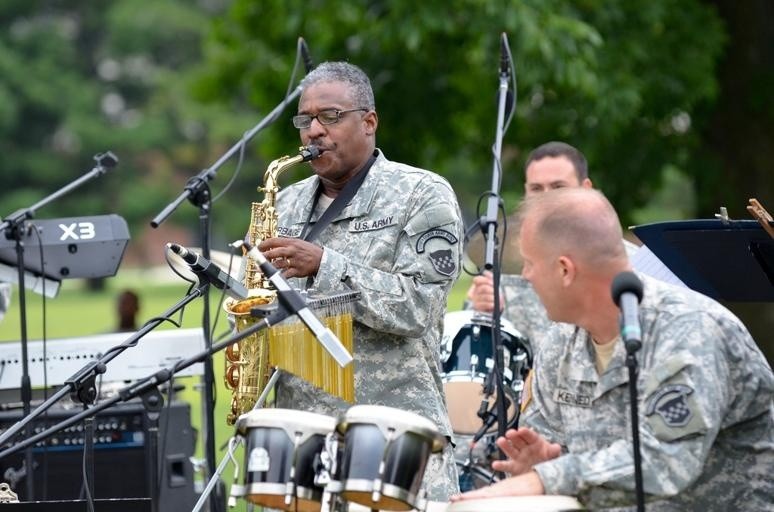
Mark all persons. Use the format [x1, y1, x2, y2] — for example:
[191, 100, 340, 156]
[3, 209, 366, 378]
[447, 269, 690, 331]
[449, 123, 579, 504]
[242, 62, 463, 503]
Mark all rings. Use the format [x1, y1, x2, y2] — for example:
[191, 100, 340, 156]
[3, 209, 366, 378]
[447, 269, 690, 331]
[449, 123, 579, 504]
[287, 260, 291, 268]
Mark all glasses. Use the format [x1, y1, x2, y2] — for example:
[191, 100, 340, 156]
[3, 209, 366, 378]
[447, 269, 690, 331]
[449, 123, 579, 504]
[292, 107, 369, 129]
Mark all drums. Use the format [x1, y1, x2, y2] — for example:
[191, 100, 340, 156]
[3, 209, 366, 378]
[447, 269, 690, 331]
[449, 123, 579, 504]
[439, 308, 535, 437]
[329, 404, 442, 511]
[235, 405, 336, 511]
[448, 494, 582, 512]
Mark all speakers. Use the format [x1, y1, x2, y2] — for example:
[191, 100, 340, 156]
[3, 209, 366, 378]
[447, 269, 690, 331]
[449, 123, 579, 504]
[0, 399, 198, 512]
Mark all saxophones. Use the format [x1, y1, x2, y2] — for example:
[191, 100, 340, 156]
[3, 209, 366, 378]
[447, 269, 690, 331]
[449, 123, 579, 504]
[222, 144, 340, 425]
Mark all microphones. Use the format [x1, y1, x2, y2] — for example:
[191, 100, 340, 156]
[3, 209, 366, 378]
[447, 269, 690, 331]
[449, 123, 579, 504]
[496, 40, 517, 114]
[471, 394, 512, 446]
[238, 238, 353, 369]
[610, 271, 646, 354]
[301, 40, 317, 72]
[168, 240, 249, 303]
